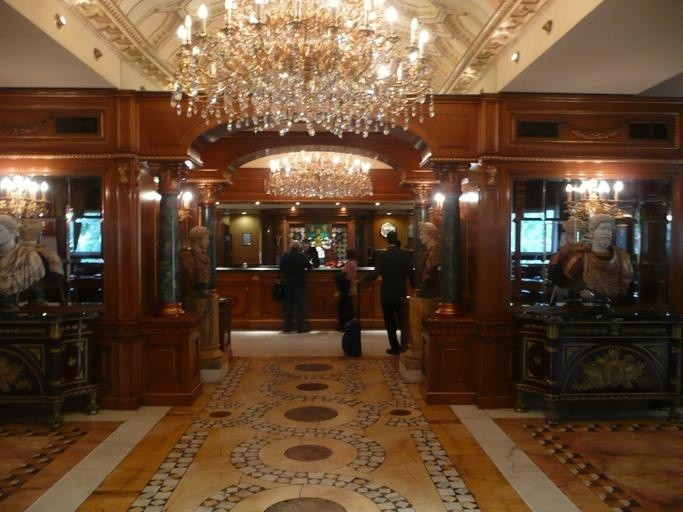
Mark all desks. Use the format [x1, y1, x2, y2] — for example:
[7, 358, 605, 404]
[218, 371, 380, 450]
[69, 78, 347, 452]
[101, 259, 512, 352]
[0, 311, 105, 429]
[512, 309, 683, 427]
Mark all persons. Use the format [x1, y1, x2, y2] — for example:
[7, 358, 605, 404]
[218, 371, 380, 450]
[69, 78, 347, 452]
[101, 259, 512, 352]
[188, 225, 212, 284]
[0, 215, 65, 296]
[333, 247, 360, 330]
[355, 231, 415, 354]
[301, 238, 318, 267]
[278, 240, 312, 333]
[550, 215, 635, 304]
[416, 220, 439, 282]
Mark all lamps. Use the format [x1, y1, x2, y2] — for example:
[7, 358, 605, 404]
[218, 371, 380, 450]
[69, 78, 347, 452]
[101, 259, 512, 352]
[169, 0, 435, 139]
[0, 174, 52, 219]
[563, 180, 639, 227]
[266, 151, 373, 199]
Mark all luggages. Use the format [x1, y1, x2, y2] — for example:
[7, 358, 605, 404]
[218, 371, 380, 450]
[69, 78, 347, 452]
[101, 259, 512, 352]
[341, 281, 362, 356]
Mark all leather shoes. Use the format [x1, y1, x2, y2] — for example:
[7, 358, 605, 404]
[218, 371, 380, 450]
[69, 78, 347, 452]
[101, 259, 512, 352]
[386, 345, 411, 355]
